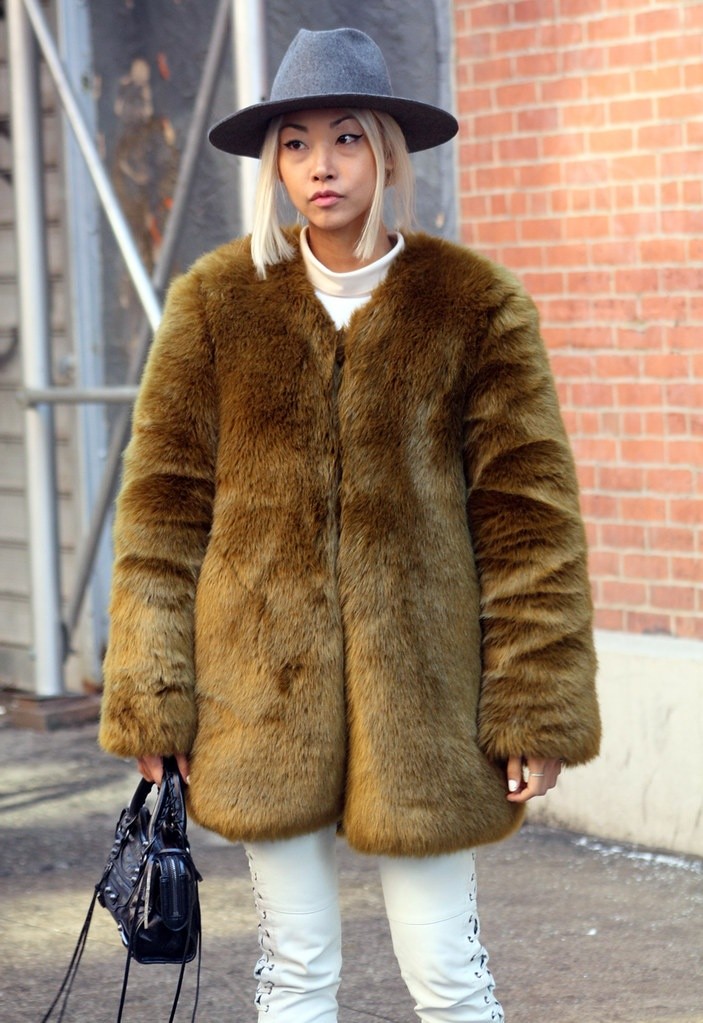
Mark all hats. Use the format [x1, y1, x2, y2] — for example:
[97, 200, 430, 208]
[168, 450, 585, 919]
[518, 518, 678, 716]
[208, 28, 459, 160]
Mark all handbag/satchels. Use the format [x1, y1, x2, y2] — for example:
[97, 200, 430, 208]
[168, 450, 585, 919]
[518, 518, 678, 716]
[41, 756, 203, 1023]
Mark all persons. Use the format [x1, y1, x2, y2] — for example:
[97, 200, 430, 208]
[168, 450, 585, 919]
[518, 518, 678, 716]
[100, 26, 599, 1023]
